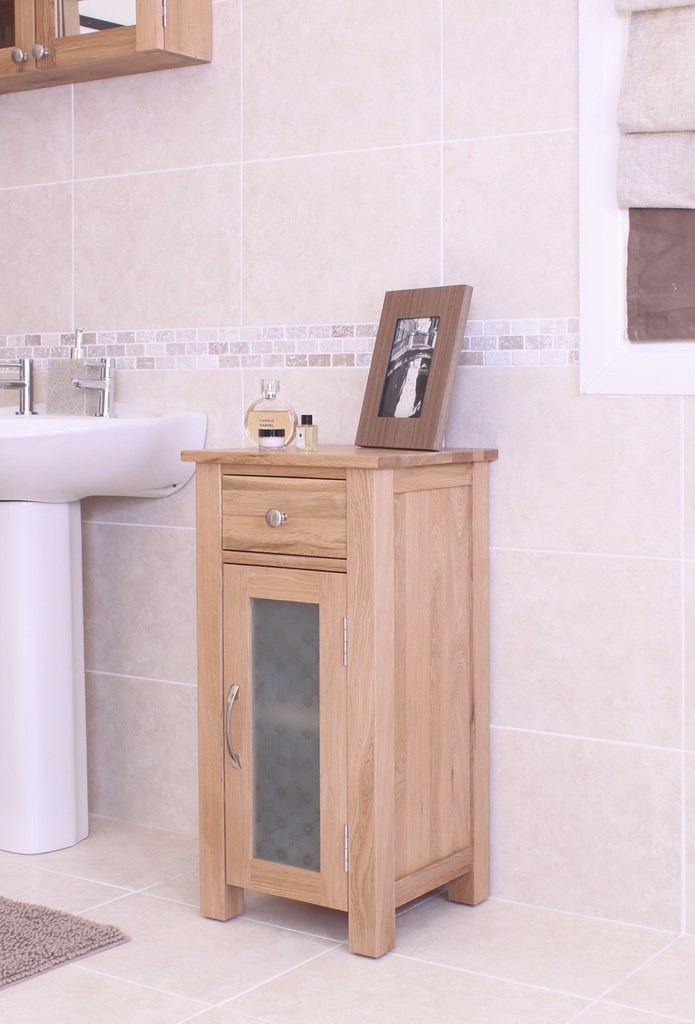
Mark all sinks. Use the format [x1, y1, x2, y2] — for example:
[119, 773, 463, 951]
[0, 412, 208, 504]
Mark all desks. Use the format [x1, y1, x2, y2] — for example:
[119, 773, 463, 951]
[179, 446, 503, 957]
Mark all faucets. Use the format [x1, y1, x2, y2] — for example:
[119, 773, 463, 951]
[71, 357, 118, 417]
[0, 358, 39, 415]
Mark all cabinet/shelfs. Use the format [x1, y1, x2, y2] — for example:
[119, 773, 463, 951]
[0, 0, 214, 95]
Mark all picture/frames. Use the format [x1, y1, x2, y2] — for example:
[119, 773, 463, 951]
[353, 283, 474, 453]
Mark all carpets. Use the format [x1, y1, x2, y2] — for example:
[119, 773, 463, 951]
[0, 896, 131, 990]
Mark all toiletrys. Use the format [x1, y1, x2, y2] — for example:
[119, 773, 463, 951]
[243, 378, 319, 453]
